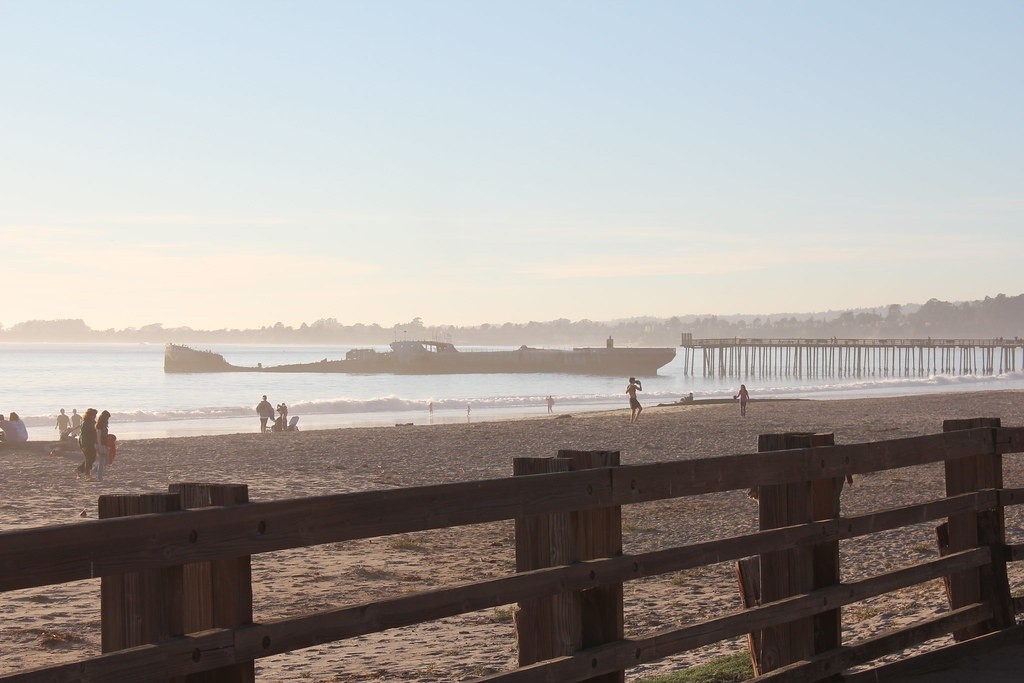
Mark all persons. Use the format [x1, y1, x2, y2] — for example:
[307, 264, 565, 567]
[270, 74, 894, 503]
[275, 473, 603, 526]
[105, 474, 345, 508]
[467, 405, 471, 414]
[429, 401, 433, 414]
[72, 407, 111, 484]
[71, 409, 81, 434]
[55, 407, 69, 439]
[0, 412, 29, 443]
[545, 395, 555, 414]
[735, 385, 750, 417]
[277, 402, 288, 429]
[256, 395, 272, 433]
[625, 378, 643, 424]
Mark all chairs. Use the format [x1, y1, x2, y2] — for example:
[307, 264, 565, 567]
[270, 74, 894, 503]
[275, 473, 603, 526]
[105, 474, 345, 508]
[265, 416, 299, 433]
